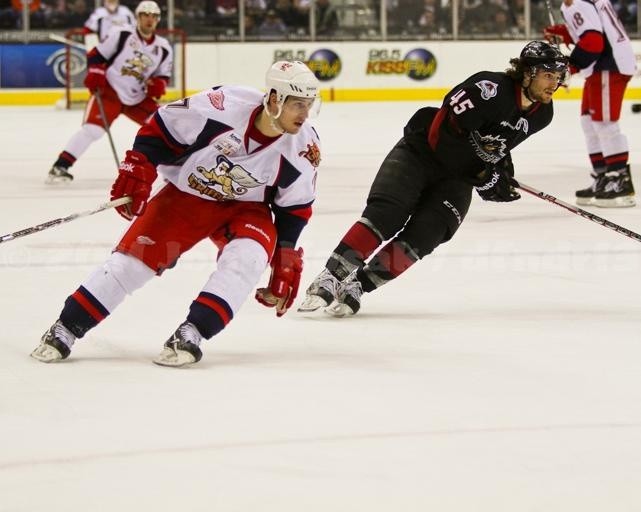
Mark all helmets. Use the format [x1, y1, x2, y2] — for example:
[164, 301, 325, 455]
[266, 60, 322, 120]
[518, 40, 570, 83]
[135, 1, 161, 25]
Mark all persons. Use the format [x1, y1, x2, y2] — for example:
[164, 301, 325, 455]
[543, 1, 638, 200]
[306, 39, 572, 315]
[39, 61, 322, 364]
[0, 0, 639, 38]
[82, 1, 136, 87]
[46, 1, 174, 181]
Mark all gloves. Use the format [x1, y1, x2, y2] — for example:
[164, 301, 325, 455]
[542, 25, 573, 45]
[146, 78, 166, 98]
[85, 68, 107, 97]
[111, 151, 158, 222]
[255, 247, 304, 318]
[473, 165, 521, 202]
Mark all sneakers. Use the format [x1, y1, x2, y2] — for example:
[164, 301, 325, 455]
[49, 159, 73, 181]
[576, 164, 635, 200]
[41, 319, 78, 360]
[166, 321, 204, 362]
[306, 267, 363, 315]
